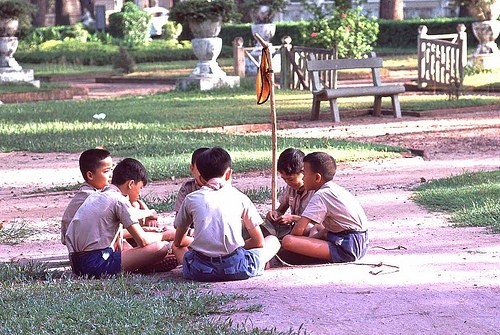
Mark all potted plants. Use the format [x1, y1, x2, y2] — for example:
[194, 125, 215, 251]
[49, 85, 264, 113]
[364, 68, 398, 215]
[467, 0, 500, 21]
[240, 0, 292, 24]
[166, 0, 243, 39]
[0, 0, 39, 37]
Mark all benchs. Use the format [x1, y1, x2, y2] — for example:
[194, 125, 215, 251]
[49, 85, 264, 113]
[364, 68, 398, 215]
[305, 52, 406, 122]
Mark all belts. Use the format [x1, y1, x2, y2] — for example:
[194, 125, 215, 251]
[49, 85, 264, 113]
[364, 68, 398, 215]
[328, 230, 366, 235]
[188, 247, 241, 261]
[69, 252, 87, 256]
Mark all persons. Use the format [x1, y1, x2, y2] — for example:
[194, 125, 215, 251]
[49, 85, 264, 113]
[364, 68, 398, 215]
[281, 151, 369, 263]
[266, 148, 315, 232]
[61, 149, 157, 247]
[65, 157, 179, 280]
[171, 146, 280, 280]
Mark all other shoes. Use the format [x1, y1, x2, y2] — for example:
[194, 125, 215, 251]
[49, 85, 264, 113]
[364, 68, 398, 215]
[277, 248, 326, 265]
[139, 254, 177, 274]
